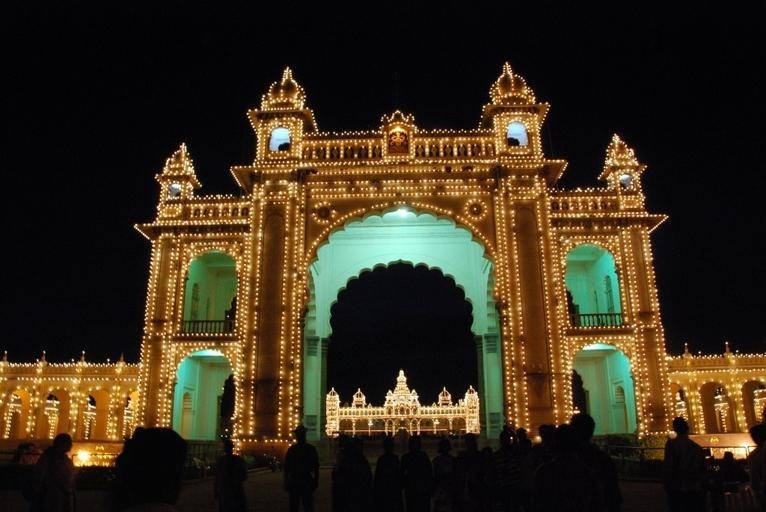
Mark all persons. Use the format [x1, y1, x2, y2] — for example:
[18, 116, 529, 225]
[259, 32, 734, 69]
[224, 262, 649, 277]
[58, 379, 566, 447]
[22, 412, 766, 512]
